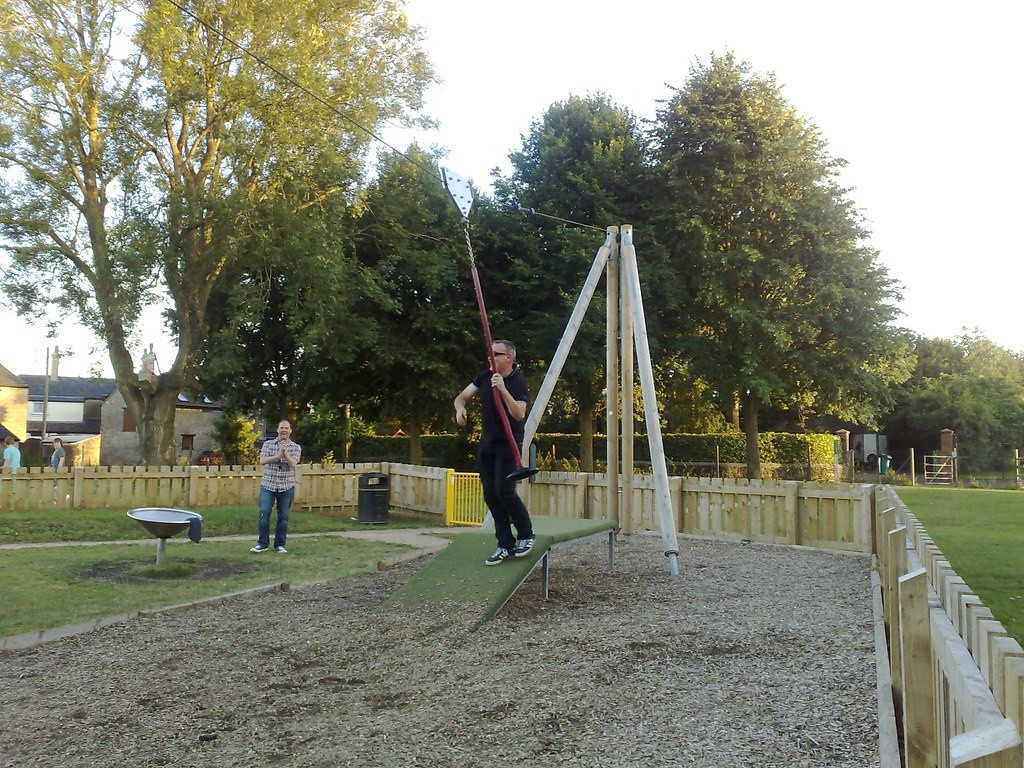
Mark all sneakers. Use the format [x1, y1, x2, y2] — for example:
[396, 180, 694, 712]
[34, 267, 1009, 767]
[276, 546, 287, 553]
[515, 530, 536, 557]
[250, 545, 268, 553]
[486, 547, 515, 566]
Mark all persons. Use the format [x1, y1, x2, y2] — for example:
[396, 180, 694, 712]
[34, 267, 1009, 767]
[50, 438, 70, 505]
[454, 338, 537, 566]
[249, 418, 301, 554]
[2, 436, 21, 511]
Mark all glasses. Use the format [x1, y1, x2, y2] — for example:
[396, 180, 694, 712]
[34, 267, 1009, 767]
[486, 351, 508, 357]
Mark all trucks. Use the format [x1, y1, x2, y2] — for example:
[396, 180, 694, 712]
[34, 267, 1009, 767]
[851, 432, 889, 471]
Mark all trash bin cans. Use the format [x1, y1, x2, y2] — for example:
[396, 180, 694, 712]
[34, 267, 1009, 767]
[357, 472, 390, 524]
[881, 454, 892, 474]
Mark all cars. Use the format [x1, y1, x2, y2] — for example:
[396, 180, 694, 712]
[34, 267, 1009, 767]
[196, 448, 221, 466]
